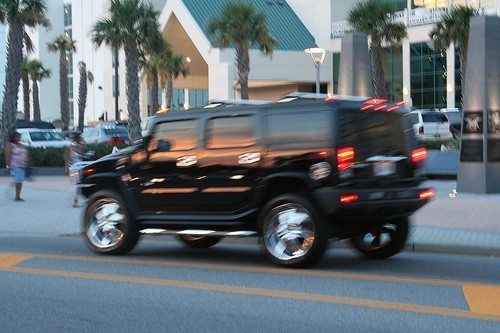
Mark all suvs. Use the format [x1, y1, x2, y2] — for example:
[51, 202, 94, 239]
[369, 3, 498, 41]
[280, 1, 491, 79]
[76, 98, 436, 267]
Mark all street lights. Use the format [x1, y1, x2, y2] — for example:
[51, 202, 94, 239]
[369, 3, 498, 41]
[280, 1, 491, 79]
[305, 47, 327, 93]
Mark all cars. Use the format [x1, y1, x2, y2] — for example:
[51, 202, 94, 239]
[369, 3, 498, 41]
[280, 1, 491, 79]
[199, 91, 372, 109]
[404, 109, 452, 146]
[15, 121, 55, 128]
[16, 129, 71, 148]
[78, 126, 131, 146]
[440, 108, 462, 130]
[96, 121, 119, 126]
